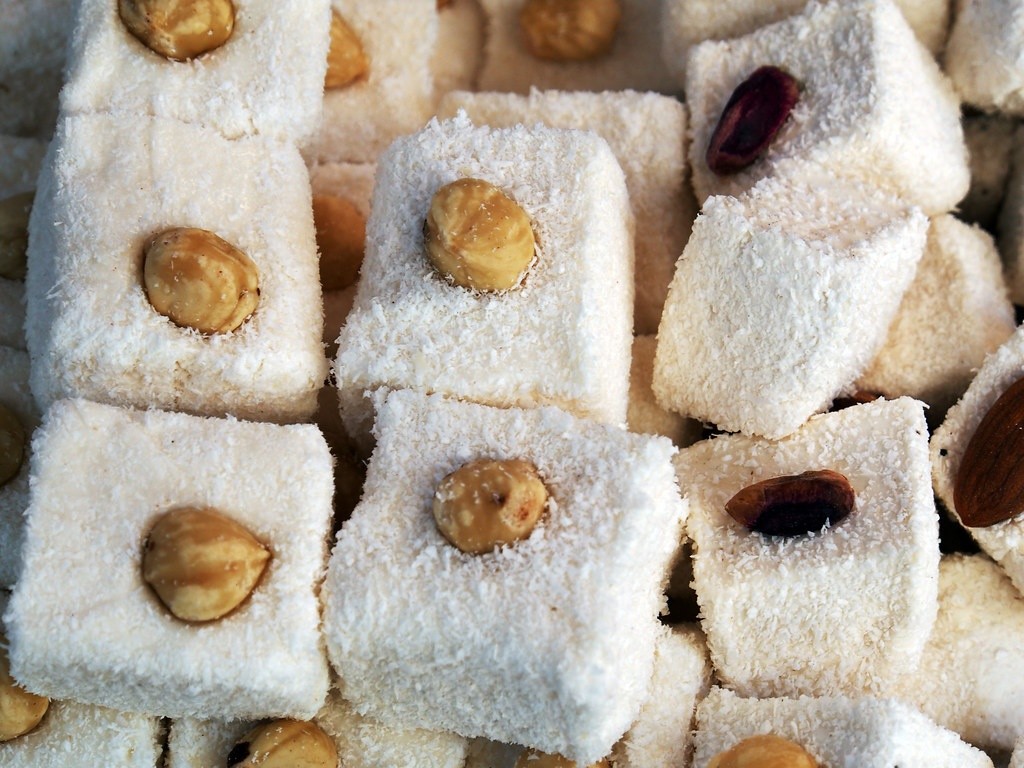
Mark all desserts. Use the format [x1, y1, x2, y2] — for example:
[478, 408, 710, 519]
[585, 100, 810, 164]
[0, 0, 1024, 768]
